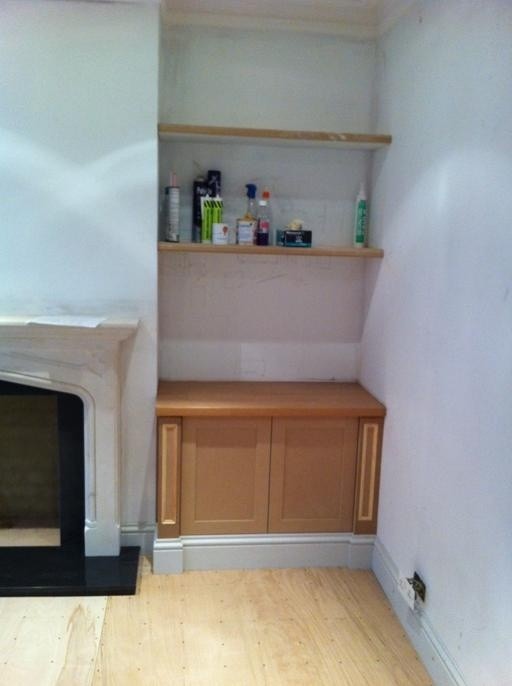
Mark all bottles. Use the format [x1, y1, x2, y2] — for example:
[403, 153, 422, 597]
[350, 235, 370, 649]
[165, 185, 182, 243]
[354, 181, 369, 249]
[192, 169, 223, 244]
[236, 183, 274, 246]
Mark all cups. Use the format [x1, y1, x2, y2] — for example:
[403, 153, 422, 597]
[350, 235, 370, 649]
[212, 223, 229, 244]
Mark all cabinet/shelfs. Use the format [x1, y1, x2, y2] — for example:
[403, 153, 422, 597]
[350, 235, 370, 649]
[156, 379, 386, 535]
[158, 123, 392, 258]
